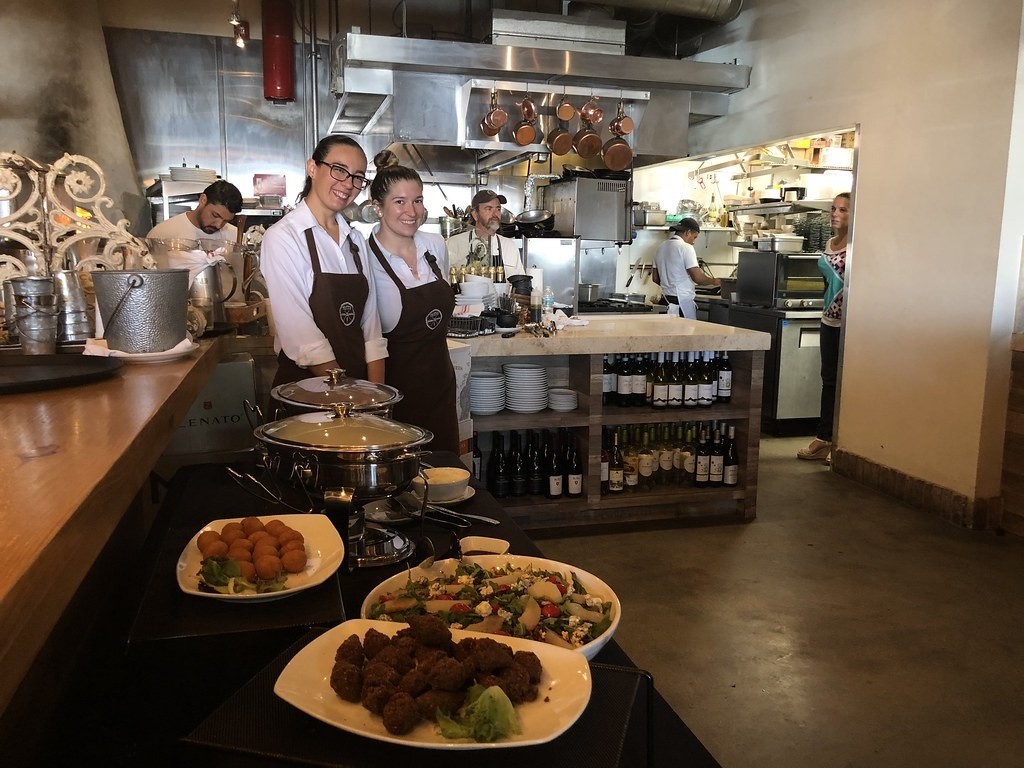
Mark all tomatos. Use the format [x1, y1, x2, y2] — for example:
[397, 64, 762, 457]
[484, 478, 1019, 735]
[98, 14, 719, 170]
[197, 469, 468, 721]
[494, 631, 508, 635]
[557, 583, 567, 596]
[451, 580, 461, 584]
[543, 605, 560, 618]
[546, 577, 558, 583]
[450, 603, 471, 612]
[491, 603, 500, 614]
[436, 594, 452, 600]
[380, 595, 394, 601]
[499, 584, 510, 592]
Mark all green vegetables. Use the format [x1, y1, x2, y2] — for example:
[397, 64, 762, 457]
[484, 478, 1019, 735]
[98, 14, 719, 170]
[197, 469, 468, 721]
[436, 686, 521, 740]
[197, 556, 290, 593]
[371, 562, 612, 641]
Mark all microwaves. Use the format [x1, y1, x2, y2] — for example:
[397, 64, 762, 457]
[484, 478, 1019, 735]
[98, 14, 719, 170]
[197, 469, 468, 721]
[736, 250, 827, 308]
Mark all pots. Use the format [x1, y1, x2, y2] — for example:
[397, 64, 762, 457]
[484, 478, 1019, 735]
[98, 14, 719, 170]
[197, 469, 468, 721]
[496, 206, 552, 238]
[252, 367, 435, 510]
[485, 93, 635, 171]
[342, 199, 379, 224]
[553, 306, 574, 317]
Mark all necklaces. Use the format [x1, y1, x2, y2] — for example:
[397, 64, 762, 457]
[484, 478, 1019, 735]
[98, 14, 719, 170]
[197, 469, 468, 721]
[403, 255, 417, 271]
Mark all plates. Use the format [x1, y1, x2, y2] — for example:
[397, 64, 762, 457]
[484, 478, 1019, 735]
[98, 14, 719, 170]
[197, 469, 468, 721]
[106, 344, 199, 363]
[175, 514, 621, 747]
[410, 486, 475, 504]
[364, 499, 413, 522]
[455, 293, 578, 416]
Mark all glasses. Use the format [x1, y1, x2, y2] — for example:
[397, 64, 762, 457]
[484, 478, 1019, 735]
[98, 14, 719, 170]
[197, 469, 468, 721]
[316, 160, 368, 191]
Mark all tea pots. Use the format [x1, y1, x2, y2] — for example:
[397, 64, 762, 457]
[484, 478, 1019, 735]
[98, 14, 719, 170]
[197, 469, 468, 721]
[496, 313, 519, 328]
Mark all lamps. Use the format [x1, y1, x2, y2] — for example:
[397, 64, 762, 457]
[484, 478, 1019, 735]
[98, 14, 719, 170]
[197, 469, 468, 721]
[229, 0, 244, 48]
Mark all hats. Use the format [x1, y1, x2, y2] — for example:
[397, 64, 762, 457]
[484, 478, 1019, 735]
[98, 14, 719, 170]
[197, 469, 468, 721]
[472, 190, 507, 207]
[669, 218, 699, 234]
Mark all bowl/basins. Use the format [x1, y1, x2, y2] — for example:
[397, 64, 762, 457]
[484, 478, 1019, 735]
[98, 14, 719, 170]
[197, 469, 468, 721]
[460, 536, 510, 556]
[412, 467, 471, 500]
[461, 274, 511, 295]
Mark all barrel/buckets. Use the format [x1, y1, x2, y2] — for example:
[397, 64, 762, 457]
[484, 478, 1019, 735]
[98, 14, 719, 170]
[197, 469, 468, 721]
[4, 269, 190, 356]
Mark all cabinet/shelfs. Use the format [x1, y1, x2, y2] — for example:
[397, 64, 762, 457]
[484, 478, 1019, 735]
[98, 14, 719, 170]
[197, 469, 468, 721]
[446, 314, 772, 543]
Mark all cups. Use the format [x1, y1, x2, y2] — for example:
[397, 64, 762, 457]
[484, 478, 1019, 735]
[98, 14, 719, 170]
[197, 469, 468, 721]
[144, 238, 260, 308]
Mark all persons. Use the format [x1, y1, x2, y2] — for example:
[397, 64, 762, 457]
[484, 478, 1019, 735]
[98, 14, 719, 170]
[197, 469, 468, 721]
[145, 179, 244, 243]
[651, 217, 722, 320]
[259, 135, 391, 418]
[356, 146, 461, 459]
[795, 192, 851, 464]
[444, 189, 532, 307]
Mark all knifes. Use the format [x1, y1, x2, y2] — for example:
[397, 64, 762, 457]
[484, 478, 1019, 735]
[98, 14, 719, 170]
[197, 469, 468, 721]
[427, 504, 501, 524]
[626, 257, 642, 288]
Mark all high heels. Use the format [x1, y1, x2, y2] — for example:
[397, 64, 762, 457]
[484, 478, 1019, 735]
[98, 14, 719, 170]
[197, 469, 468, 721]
[826, 455, 832, 465]
[797, 438, 832, 459]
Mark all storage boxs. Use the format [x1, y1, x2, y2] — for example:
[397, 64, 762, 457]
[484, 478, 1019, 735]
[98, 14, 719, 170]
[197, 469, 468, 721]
[446, 338, 473, 474]
[760, 135, 842, 165]
[480, 9, 627, 54]
[162, 352, 259, 457]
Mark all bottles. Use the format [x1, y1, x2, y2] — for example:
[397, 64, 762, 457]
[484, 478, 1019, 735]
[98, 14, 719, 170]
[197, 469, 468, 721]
[470, 420, 739, 498]
[529, 286, 556, 324]
[708, 193, 733, 227]
[449, 264, 504, 294]
[602, 351, 732, 408]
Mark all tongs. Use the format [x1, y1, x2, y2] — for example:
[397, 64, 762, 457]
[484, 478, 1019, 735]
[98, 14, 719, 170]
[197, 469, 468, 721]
[384, 491, 473, 529]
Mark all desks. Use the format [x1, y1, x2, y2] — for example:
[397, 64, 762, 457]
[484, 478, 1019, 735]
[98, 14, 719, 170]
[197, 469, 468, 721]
[33, 450, 724, 768]
